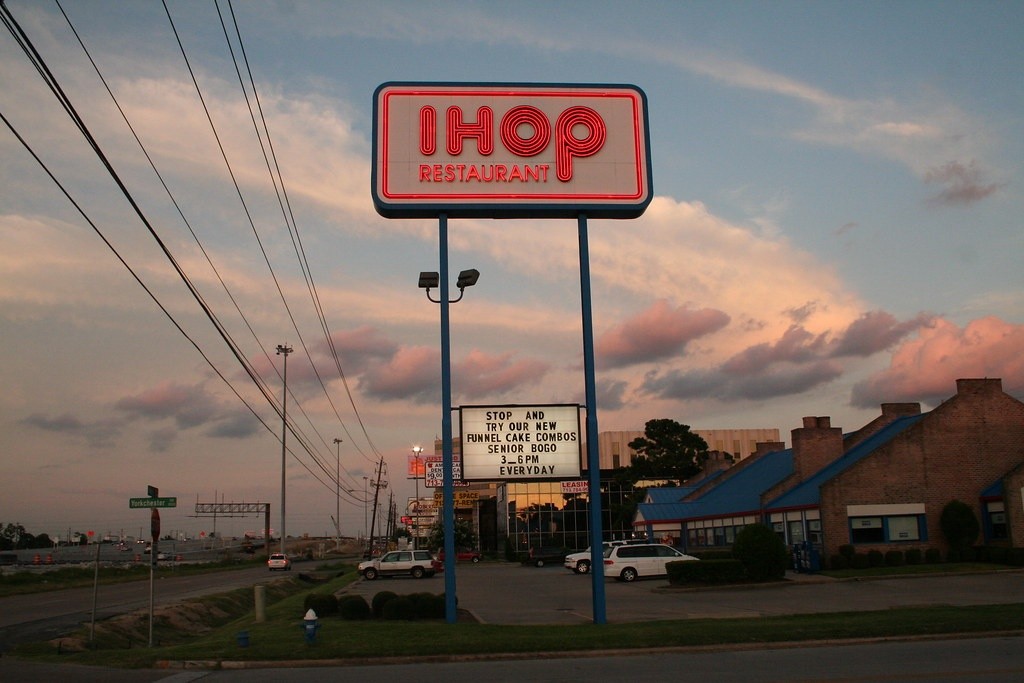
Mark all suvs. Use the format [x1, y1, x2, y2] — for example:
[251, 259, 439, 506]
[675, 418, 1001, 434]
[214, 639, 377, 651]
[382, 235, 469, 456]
[357, 548, 436, 579]
[268, 554, 291, 571]
[563, 539, 651, 573]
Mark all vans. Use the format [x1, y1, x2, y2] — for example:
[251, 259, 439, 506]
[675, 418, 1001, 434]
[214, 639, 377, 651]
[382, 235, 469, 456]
[601, 544, 701, 582]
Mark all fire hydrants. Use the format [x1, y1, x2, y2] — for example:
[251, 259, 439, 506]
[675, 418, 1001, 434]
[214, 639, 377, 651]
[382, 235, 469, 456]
[302, 609, 320, 644]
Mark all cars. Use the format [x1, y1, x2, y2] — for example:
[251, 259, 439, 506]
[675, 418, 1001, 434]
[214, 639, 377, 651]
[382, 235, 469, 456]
[137, 539, 144, 544]
[121, 546, 133, 552]
[528, 544, 575, 567]
[157, 552, 173, 561]
[184, 538, 192, 542]
[437, 546, 483, 562]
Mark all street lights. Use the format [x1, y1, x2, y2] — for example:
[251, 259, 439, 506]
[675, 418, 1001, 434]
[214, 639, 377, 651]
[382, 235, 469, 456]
[413, 444, 424, 549]
[363, 476, 369, 550]
[332, 437, 343, 552]
[348, 489, 381, 548]
[273, 343, 293, 553]
